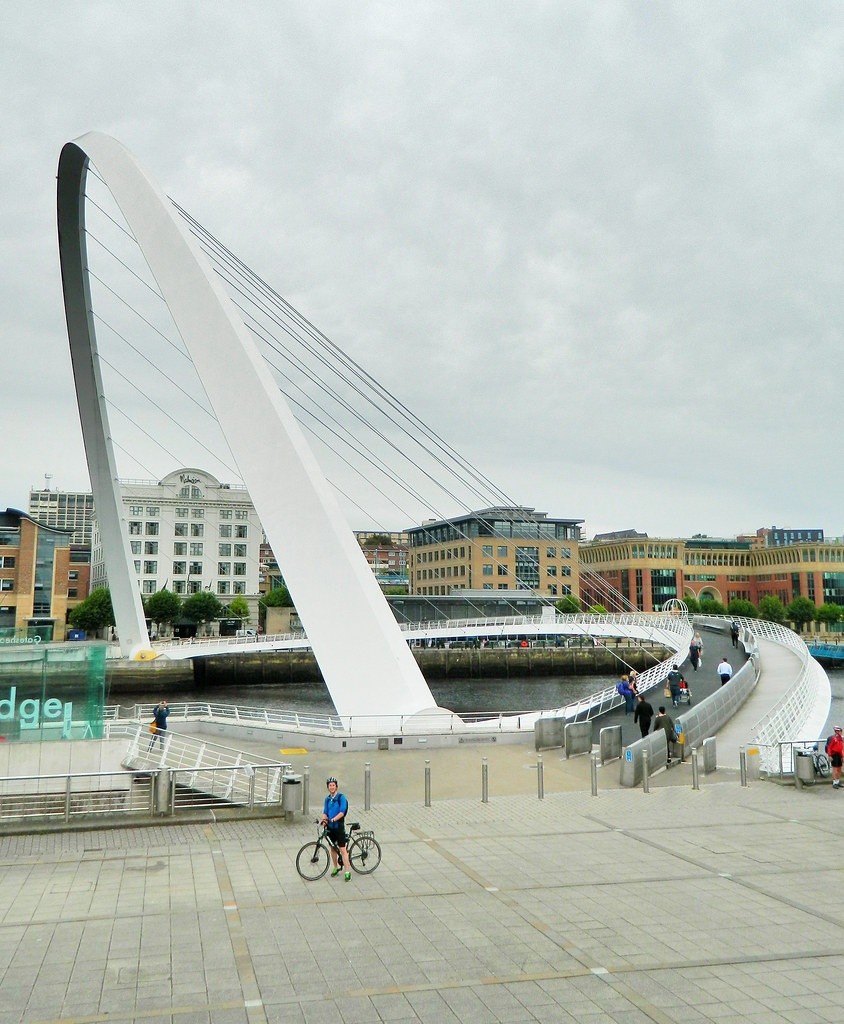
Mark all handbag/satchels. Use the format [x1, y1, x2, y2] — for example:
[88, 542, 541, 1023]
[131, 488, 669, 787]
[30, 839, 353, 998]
[736, 632, 740, 637]
[698, 658, 702, 668]
[670, 729, 677, 744]
[663, 687, 672, 697]
[149, 722, 157, 733]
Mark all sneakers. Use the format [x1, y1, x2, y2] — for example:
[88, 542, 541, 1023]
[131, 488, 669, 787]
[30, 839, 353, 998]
[344, 871, 352, 882]
[331, 866, 341, 877]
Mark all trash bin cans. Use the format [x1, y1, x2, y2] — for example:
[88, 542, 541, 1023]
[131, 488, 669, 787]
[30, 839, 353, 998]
[793, 747, 814, 789]
[282, 770, 303, 822]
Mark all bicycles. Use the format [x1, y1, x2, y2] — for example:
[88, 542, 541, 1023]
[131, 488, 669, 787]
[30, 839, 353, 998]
[296, 819, 382, 881]
[803, 745, 832, 778]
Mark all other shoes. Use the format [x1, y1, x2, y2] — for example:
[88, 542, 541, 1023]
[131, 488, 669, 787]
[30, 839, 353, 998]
[833, 783, 844, 789]
[668, 758, 671, 763]
[672, 701, 678, 708]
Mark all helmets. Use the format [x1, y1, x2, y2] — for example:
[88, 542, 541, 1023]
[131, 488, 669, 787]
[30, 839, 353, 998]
[833, 726, 842, 732]
[327, 777, 337, 783]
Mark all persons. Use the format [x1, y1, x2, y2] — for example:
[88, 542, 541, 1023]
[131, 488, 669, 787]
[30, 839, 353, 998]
[147, 701, 170, 753]
[621, 671, 639, 715]
[322, 776, 352, 882]
[717, 657, 733, 686]
[667, 664, 684, 708]
[730, 622, 739, 648]
[689, 633, 703, 672]
[825, 726, 844, 789]
[634, 695, 654, 738]
[483, 639, 490, 648]
[254, 625, 260, 643]
[654, 706, 675, 763]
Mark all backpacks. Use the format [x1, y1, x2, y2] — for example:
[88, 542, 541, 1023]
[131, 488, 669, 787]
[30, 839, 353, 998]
[825, 736, 832, 753]
[618, 683, 626, 695]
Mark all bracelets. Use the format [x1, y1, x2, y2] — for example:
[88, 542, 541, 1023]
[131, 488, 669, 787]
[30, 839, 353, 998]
[332, 818, 334, 822]
